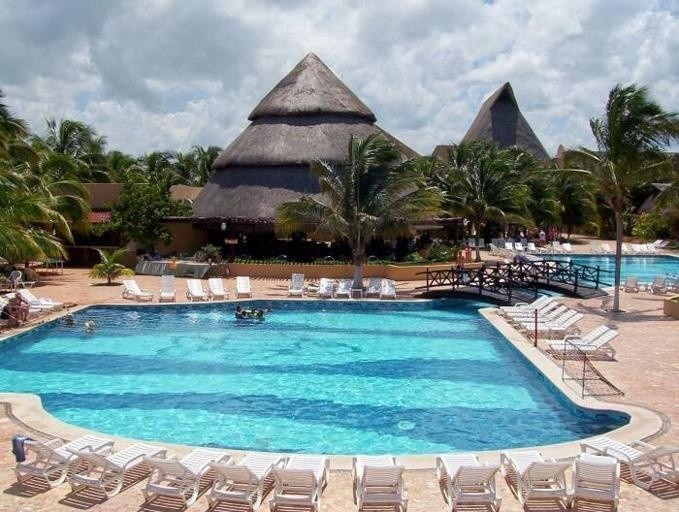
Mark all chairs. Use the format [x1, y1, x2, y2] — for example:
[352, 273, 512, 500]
[13, 436, 116, 490]
[206, 454, 289, 511]
[351, 454, 409, 511]
[142, 448, 234, 509]
[68, 442, 168, 500]
[122, 273, 398, 302]
[500, 451, 569, 511]
[437, 455, 502, 511]
[465, 234, 669, 261]
[580, 435, 678, 491]
[626, 274, 679, 294]
[500, 294, 621, 362]
[1, 270, 65, 321]
[268, 457, 331, 510]
[568, 454, 621, 511]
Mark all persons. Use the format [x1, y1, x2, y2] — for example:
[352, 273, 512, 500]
[0, 292, 29, 328]
[539, 229, 546, 245]
[8, 267, 22, 293]
[456, 245, 472, 284]
[235, 306, 264, 319]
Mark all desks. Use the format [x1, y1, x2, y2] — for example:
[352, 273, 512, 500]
[136, 260, 233, 280]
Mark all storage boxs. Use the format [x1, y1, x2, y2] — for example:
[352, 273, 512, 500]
[663, 295, 679, 321]
